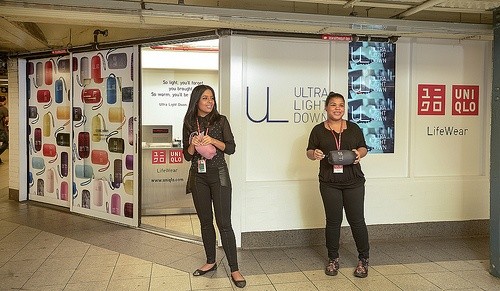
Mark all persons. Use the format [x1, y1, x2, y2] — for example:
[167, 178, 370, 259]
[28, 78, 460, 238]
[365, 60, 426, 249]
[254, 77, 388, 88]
[306, 91, 370, 278]
[0, 96, 9, 165]
[182, 85, 247, 288]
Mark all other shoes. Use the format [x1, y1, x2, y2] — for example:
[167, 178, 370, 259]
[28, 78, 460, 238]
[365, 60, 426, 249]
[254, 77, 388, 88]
[231, 273, 246, 287]
[193, 263, 217, 276]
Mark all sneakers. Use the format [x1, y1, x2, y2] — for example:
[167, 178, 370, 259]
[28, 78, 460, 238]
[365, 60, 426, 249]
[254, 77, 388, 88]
[354, 259, 368, 278]
[325, 259, 339, 276]
